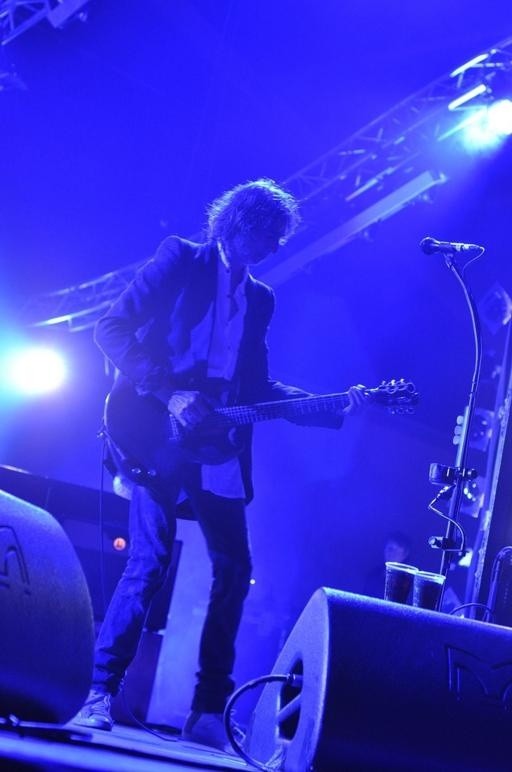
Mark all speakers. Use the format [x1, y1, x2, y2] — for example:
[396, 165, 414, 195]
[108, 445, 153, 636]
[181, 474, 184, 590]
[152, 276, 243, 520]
[242, 586, 512, 772]
[0, 490, 96, 726]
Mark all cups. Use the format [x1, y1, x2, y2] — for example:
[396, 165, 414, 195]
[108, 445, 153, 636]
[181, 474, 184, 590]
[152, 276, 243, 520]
[413, 568, 447, 609]
[383, 561, 418, 603]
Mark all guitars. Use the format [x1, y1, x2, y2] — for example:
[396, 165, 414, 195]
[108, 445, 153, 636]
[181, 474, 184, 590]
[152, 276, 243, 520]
[104, 377, 418, 478]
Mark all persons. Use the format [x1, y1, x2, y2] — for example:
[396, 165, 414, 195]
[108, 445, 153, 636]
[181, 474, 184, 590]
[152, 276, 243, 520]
[69, 180, 371, 755]
[364, 531, 415, 606]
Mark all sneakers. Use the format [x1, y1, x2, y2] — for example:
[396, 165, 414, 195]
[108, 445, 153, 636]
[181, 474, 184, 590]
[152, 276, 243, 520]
[181, 709, 248, 756]
[72, 688, 113, 731]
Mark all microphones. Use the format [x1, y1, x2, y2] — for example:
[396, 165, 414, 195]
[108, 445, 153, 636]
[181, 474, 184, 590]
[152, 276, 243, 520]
[419, 237, 481, 255]
